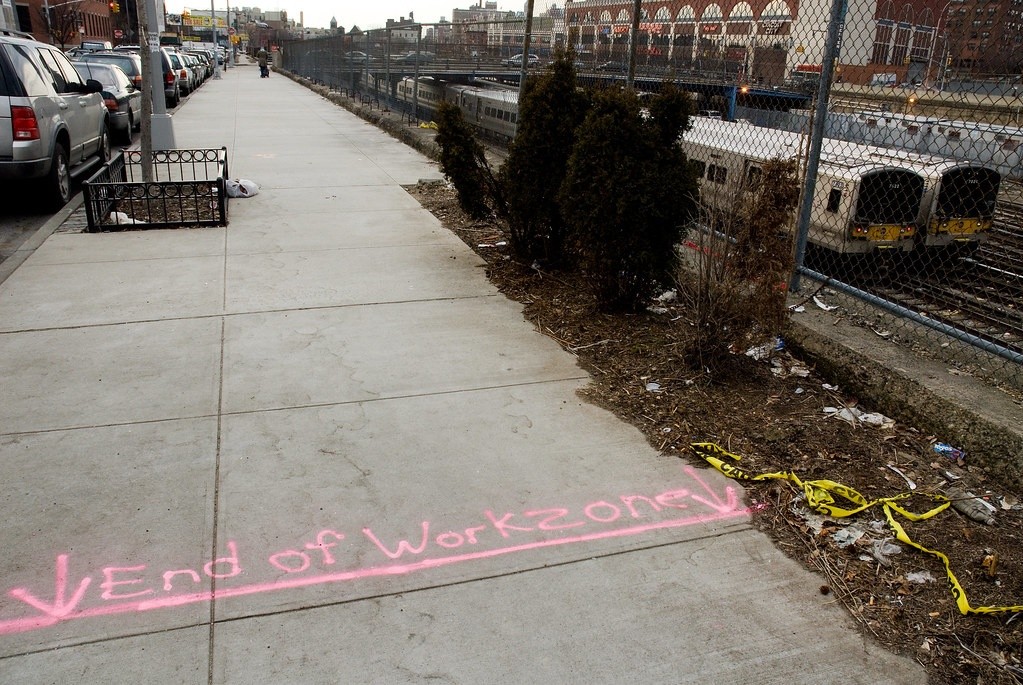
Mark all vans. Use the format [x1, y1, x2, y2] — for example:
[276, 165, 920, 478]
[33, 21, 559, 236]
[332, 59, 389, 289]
[784, 70, 821, 88]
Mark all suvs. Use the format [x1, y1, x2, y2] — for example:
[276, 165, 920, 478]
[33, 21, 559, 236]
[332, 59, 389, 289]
[0, 28, 111, 208]
[80, 48, 143, 90]
[501, 53, 541, 69]
[113, 44, 183, 109]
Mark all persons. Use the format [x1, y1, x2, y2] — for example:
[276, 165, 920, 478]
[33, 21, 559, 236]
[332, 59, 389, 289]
[256, 47, 268, 78]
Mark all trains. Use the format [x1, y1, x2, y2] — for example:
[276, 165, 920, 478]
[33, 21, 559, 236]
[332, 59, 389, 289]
[360, 76, 1001, 285]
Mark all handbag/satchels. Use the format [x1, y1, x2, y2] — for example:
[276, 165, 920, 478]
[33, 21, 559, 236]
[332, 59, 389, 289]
[224, 177, 259, 197]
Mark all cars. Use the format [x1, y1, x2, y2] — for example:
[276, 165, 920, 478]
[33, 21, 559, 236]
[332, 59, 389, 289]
[67, 60, 142, 146]
[158, 41, 230, 97]
[548, 55, 585, 73]
[595, 60, 628, 73]
[395, 53, 434, 67]
[341, 50, 374, 66]
